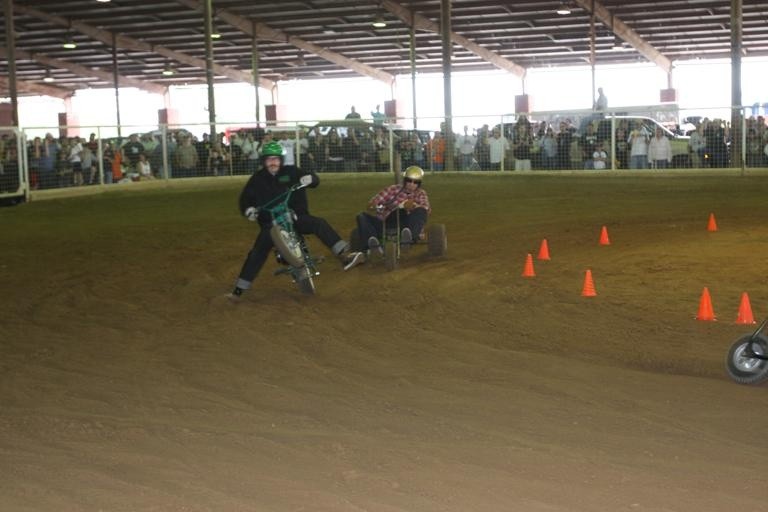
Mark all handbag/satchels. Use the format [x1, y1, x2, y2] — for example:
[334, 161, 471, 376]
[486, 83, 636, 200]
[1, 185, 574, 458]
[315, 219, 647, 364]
[78, 152, 86, 161]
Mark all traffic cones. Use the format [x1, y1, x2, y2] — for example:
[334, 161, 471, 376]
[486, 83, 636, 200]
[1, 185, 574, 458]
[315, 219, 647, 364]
[735, 289, 760, 326]
[535, 237, 551, 260]
[598, 225, 610, 247]
[692, 285, 718, 323]
[520, 251, 534, 281]
[704, 213, 717, 232]
[581, 269, 597, 299]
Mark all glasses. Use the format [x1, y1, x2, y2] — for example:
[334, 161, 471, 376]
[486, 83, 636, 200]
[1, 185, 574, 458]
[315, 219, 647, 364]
[404, 178, 419, 183]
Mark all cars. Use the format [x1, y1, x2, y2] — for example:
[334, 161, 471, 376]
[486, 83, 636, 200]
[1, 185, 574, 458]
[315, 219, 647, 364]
[470, 117, 699, 169]
[140, 128, 197, 157]
[392, 129, 437, 148]
[264, 127, 310, 156]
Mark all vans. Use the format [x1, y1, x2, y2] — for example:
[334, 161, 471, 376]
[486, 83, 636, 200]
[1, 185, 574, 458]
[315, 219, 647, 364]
[307, 119, 388, 144]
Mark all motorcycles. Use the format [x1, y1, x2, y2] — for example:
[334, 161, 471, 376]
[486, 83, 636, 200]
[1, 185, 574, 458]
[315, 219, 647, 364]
[246, 181, 326, 297]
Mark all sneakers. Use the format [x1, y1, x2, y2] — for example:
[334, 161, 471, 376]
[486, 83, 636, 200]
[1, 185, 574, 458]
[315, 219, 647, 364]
[368, 237, 384, 259]
[232, 288, 242, 295]
[400, 227, 412, 253]
[343, 252, 362, 271]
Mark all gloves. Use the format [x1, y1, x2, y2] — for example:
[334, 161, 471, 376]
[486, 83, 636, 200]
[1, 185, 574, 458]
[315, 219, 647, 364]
[245, 206, 259, 222]
[300, 175, 312, 185]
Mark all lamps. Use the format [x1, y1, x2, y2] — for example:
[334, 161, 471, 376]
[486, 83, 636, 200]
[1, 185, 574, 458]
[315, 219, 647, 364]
[60, 16, 77, 51]
[294, 49, 307, 67]
[370, 0, 386, 28]
[556, 0, 573, 16]
[211, 22, 222, 39]
[161, 59, 175, 76]
[42, 66, 56, 84]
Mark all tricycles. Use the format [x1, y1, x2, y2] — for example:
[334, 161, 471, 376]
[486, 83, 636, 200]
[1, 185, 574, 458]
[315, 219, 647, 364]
[350, 198, 448, 272]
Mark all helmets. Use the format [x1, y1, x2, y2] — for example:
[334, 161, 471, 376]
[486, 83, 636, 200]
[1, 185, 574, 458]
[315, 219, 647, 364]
[403, 166, 424, 181]
[258, 142, 287, 157]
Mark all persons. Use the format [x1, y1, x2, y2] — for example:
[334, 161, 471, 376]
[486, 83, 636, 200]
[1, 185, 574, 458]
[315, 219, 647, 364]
[223, 140, 364, 304]
[370, 104, 385, 127]
[299, 127, 390, 173]
[398, 123, 445, 171]
[615, 119, 673, 170]
[596, 87, 606, 115]
[344, 106, 361, 119]
[0, 116, 295, 190]
[355, 166, 431, 258]
[455, 119, 611, 170]
[687, 118, 768, 167]
